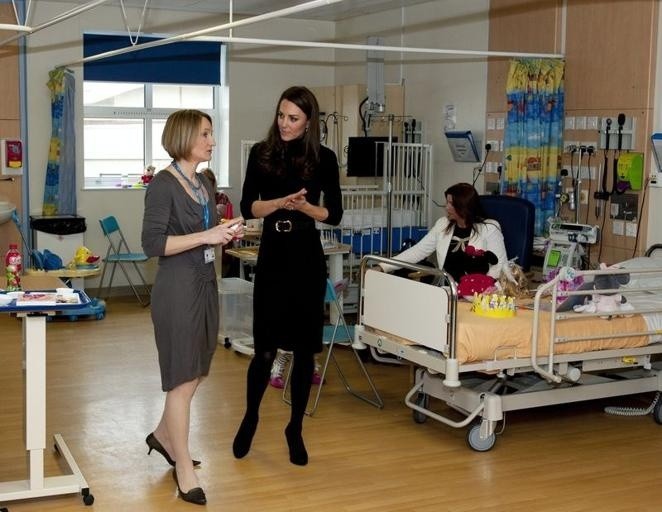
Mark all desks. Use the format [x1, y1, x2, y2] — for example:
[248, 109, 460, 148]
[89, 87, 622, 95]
[223, 240, 351, 326]
[0, 274, 93, 512]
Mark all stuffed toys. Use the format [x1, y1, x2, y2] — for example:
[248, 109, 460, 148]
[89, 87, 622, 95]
[141, 164, 156, 186]
[73, 246, 99, 266]
[574, 263, 635, 320]
[456, 244, 499, 302]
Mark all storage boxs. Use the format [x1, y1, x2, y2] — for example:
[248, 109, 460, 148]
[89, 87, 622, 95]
[215, 276, 255, 346]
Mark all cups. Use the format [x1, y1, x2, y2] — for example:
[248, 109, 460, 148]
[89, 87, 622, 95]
[245, 219, 263, 234]
[441, 104, 458, 132]
[55, 287, 75, 303]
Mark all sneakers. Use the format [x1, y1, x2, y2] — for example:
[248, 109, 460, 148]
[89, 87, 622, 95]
[268, 377, 285, 388]
[312, 373, 326, 385]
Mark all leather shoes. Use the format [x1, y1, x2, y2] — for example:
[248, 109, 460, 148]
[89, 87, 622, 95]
[286, 423, 307, 465]
[233, 414, 258, 458]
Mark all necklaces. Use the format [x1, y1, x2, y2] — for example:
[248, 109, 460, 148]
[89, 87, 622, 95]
[170, 161, 202, 190]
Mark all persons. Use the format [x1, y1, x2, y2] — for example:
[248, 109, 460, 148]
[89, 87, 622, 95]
[270, 281, 348, 389]
[233, 84, 344, 466]
[370, 181, 511, 295]
[137, 110, 246, 506]
[197, 167, 232, 265]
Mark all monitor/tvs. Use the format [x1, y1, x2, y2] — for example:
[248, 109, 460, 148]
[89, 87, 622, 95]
[346, 136, 398, 178]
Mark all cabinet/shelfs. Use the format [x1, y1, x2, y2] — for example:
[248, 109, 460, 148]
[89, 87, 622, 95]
[307, 84, 404, 209]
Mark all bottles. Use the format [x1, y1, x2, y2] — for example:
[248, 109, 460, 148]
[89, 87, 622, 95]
[5, 243, 24, 291]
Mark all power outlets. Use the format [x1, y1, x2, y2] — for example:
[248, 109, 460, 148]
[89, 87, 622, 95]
[485, 118, 505, 174]
[561, 115, 598, 180]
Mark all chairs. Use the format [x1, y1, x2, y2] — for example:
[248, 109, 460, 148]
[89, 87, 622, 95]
[283, 278, 383, 416]
[96, 216, 151, 308]
[479, 195, 535, 273]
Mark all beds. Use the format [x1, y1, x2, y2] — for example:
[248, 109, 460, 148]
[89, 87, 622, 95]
[236, 142, 432, 314]
[361, 242, 662, 451]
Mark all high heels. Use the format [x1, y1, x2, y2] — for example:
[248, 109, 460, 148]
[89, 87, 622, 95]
[146, 432, 201, 467]
[172, 468, 206, 504]
